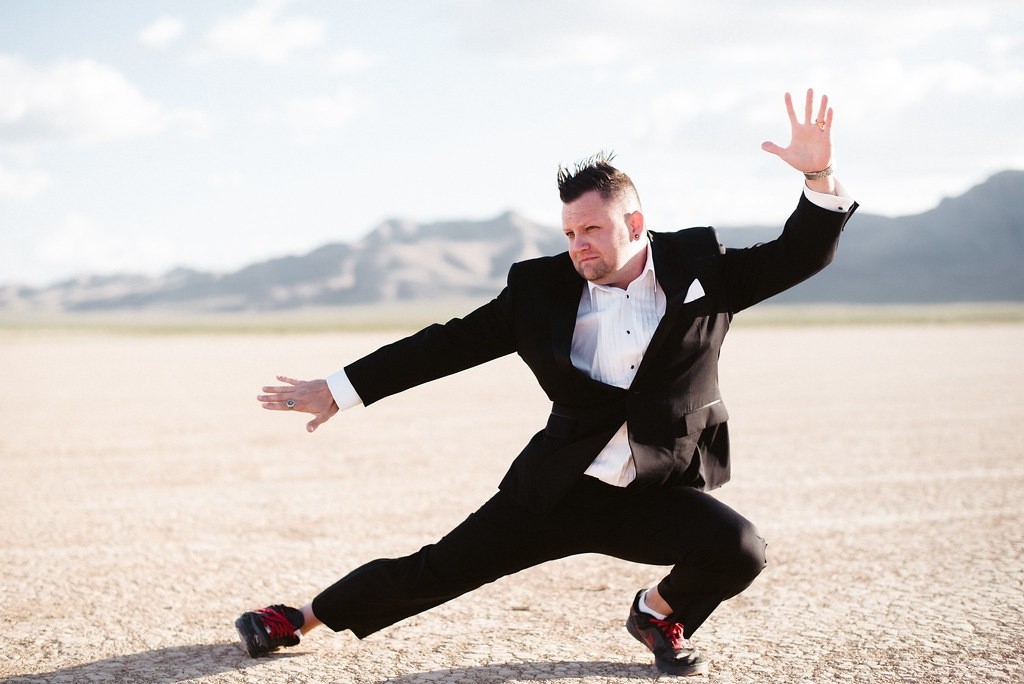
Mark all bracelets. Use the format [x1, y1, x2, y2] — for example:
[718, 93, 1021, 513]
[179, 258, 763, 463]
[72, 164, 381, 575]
[804, 164, 834, 181]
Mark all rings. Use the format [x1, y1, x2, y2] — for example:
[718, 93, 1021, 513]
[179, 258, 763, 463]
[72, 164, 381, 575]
[285, 401, 297, 411]
[815, 119, 825, 126]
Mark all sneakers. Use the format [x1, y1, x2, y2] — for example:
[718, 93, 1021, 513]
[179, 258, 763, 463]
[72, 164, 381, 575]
[235, 604, 304, 658]
[626, 588, 709, 676]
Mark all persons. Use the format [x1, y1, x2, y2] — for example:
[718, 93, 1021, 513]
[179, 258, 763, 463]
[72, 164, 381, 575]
[233, 87, 860, 675]
